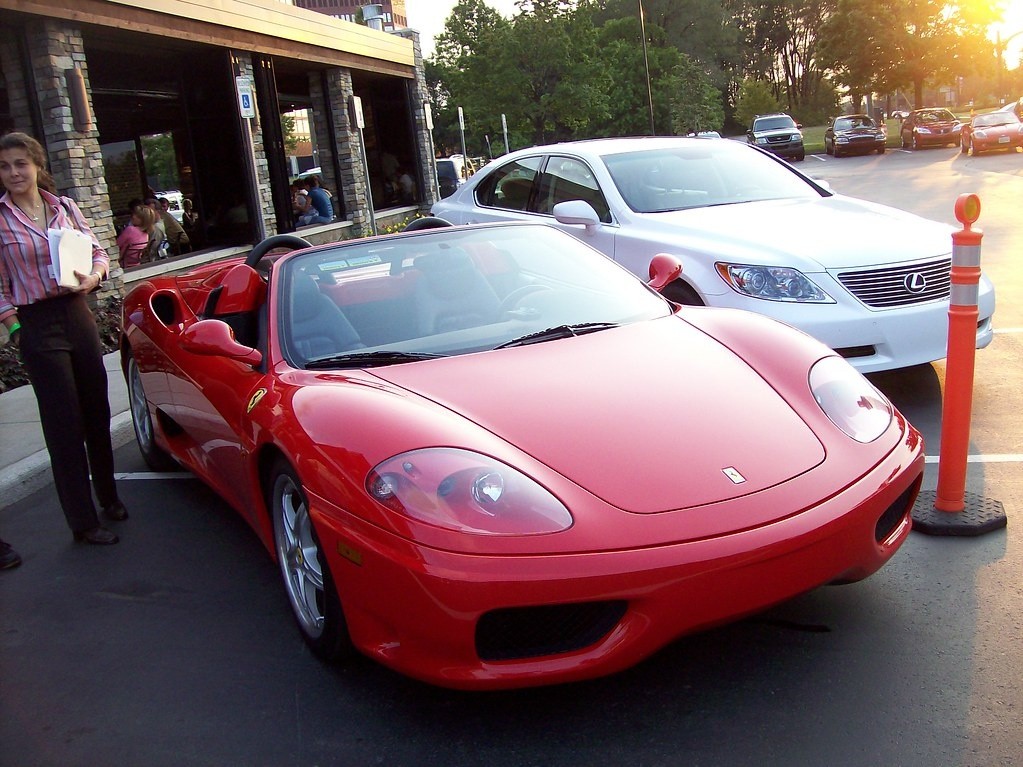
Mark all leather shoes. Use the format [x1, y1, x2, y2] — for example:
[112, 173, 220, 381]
[100, 496, 128, 521]
[72, 526, 120, 545]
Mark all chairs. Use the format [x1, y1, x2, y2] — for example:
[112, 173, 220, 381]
[409, 248, 505, 339]
[119, 231, 185, 269]
[500, 177, 534, 208]
[260, 272, 371, 378]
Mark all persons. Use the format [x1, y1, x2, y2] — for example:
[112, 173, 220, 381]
[290, 175, 334, 226]
[372, 150, 413, 206]
[0, 132, 131, 548]
[115, 197, 198, 270]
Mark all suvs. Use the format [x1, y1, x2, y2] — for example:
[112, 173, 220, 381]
[746, 112, 806, 161]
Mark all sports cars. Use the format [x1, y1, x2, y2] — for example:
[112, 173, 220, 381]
[118, 211, 928, 697]
[960, 111, 1023, 156]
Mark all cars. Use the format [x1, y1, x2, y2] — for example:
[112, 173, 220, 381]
[823, 114, 887, 157]
[685, 131, 720, 139]
[995, 101, 1017, 113]
[428, 134, 996, 376]
[901, 107, 962, 150]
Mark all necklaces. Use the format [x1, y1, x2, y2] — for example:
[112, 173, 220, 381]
[14, 199, 44, 221]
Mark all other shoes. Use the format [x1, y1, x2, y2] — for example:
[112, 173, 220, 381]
[0, 541, 22, 569]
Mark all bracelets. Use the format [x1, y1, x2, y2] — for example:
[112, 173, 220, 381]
[8, 323, 21, 341]
[95, 272, 104, 288]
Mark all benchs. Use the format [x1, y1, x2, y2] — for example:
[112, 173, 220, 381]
[544, 172, 607, 223]
